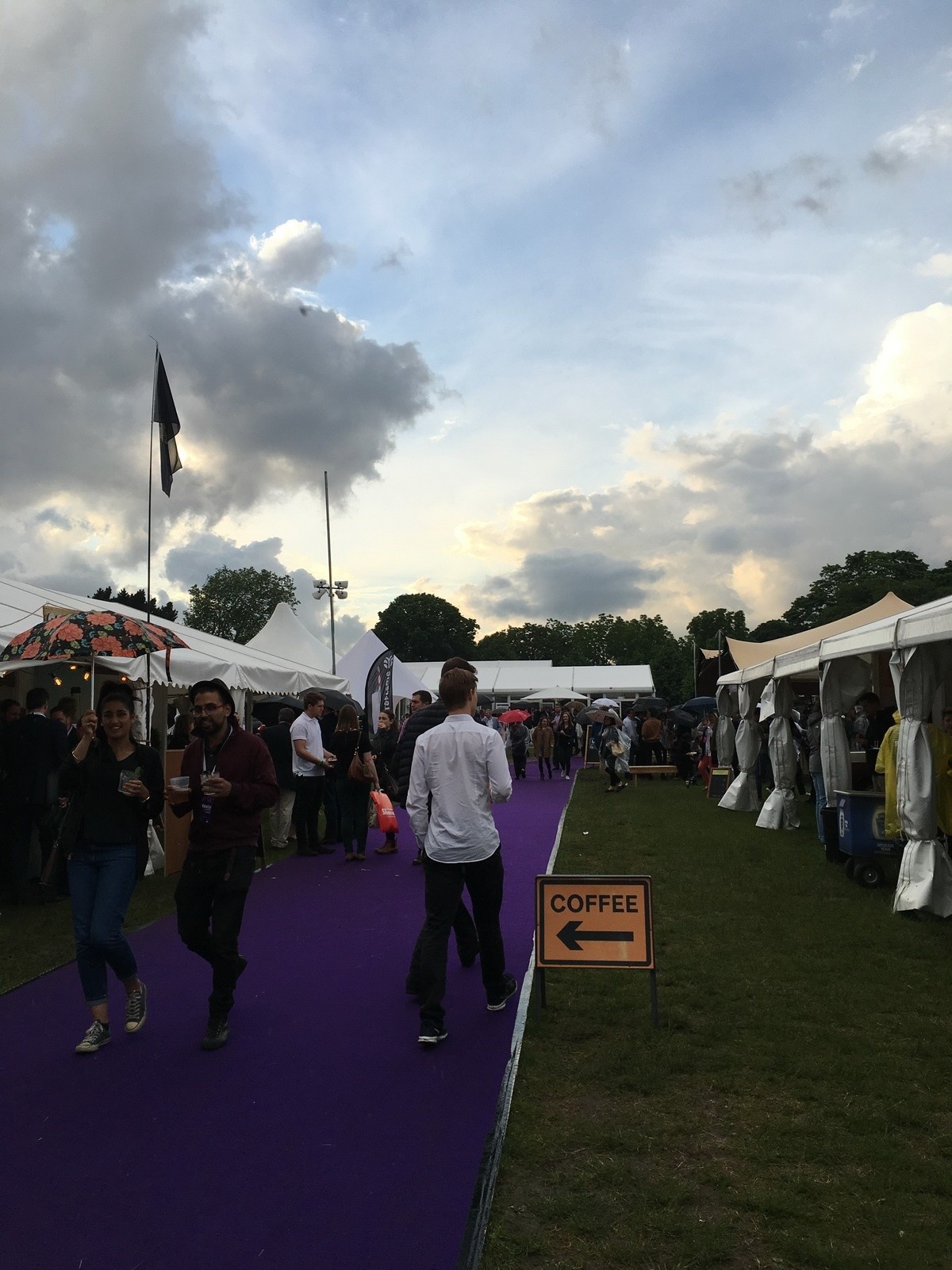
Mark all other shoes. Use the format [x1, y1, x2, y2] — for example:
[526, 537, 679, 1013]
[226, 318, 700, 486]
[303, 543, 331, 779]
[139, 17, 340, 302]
[298, 845, 318, 856]
[514, 767, 570, 781]
[345, 853, 356, 862]
[413, 857, 422, 865]
[311, 846, 334, 854]
[702, 787, 707, 791]
[604, 783, 626, 793]
[358, 854, 366, 860]
[462, 941, 480, 967]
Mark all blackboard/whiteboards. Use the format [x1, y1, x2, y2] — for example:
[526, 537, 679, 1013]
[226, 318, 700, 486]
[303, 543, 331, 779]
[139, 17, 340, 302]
[256, 823, 266, 870]
[707, 767, 730, 799]
[619, 699, 636, 731]
[584, 725, 600, 769]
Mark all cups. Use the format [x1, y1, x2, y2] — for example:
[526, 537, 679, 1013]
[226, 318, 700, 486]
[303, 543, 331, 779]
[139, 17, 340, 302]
[201, 772, 221, 794]
[170, 776, 189, 803]
[118, 769, 142, 794]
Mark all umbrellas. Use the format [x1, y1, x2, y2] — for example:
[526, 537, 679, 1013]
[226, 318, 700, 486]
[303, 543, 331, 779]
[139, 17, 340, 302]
[0, 609, 191, 739]
[477, 685, 718, 726]
[299, 687, 365, 717]
[252, 695, 305, 711]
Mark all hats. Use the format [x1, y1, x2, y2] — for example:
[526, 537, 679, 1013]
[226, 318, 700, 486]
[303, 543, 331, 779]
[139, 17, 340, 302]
[190, 679, 234, 720]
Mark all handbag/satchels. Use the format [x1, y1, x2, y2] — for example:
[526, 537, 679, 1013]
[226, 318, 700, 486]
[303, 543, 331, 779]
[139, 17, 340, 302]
[610, 727, 625, 756]
[348, 752, 374, 782]
[384, 768, 400, 801]
[42, 841, 66, 889]
[368, 788, 399, 833]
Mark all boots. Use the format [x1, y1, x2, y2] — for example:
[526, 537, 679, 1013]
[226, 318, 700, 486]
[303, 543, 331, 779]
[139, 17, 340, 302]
[374, 838, 397, 854]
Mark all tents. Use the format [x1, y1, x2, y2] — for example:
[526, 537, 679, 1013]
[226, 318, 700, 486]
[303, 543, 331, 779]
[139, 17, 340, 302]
[245, 602, 343, 673]
[713, 596, 952, 918]
[330, 629, 438, 715]
[0, 579, 351, 741]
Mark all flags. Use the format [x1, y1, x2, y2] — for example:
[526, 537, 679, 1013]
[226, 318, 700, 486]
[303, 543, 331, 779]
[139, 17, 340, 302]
[152, 348, 182, 497]
[364, 648, 396, 740]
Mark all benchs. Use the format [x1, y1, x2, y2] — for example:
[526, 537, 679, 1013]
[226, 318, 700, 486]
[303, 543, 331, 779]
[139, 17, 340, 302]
[627, 764, 678, 788]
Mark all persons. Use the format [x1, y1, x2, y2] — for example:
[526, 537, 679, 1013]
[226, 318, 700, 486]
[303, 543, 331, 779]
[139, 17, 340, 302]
[252, 690, 434, 865]
[0, 688, 92, 901]
[54, 682, 165, 1052]
[162, 679, 281, 1052]
[167, 715, 197, 749]
[732, 692, 952, 849]
[405, 669, 517, 1043]
[394, 657, 482, 993]
[477, 702, 719, 792]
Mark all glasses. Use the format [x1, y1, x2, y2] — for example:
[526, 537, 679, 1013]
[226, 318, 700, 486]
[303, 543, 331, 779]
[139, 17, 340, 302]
[191, 706, 225, 718]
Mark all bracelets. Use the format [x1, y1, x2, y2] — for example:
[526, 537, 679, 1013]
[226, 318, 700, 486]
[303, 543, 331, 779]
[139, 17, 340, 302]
[72, 751, 82, 761]
[140, 796, 150, 803]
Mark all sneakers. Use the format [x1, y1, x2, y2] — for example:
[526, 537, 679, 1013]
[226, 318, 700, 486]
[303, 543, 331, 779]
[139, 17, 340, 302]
[75, 1020, 111, 1052]
[487, 974, 516, 1011]
[201, 1016, 229, 1048]
[124, 982, 146, 1032]
[236, 955, 247, 977]
[418, 1021, 449, 1043]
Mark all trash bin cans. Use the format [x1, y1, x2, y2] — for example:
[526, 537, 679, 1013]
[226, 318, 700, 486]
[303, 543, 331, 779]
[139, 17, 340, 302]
[821, 807, 839, 864]
